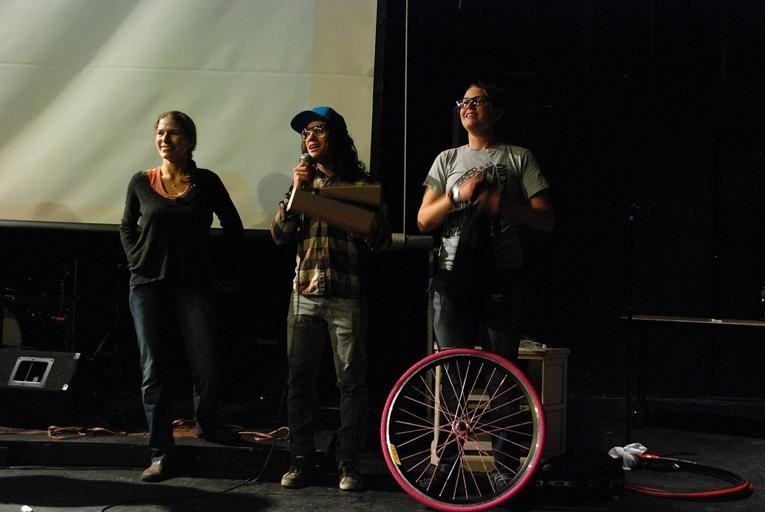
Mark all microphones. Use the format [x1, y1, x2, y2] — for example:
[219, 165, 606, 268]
[300, 153, 312, 190]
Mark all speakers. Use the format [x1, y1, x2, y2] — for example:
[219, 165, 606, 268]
[0, 348, 95, 431]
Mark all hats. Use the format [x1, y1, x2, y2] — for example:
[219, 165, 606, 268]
[291, 105, 347, 134]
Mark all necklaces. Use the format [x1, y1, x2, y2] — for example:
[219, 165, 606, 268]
[171, 184, 176, 188]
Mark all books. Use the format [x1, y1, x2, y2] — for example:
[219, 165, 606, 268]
[316, 184, 382, 209]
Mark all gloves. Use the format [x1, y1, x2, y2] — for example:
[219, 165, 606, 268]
[608, 442, 648, 471]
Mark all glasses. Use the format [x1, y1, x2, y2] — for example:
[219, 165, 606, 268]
[301, 125, 329, 141]
[455, 95, 488, 107]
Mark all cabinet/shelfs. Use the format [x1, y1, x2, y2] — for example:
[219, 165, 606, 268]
[431, 342, 571, 472]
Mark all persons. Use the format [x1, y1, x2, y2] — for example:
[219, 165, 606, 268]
[413, 85, 556, 498]
[268, 106, 388, 492]
[117, 109, 253, 482]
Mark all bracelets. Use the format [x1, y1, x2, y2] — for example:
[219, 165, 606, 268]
[447, 191, 459, 209]
[451, 187, 461, 202]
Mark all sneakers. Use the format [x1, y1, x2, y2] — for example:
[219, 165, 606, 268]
[281, 458, 305, 488]
[142, 461, 171, 481]
[207, 425, 242, 444]
[439, 461, 457, 481]
[337, 461, 359, 490]
[494, 465, 513, 488]
